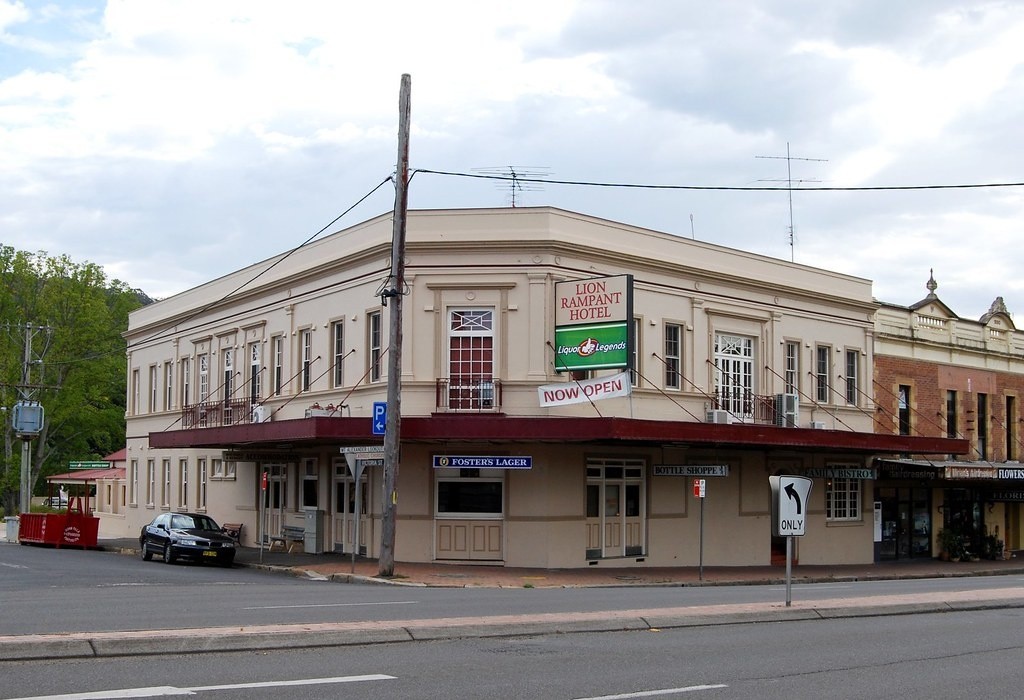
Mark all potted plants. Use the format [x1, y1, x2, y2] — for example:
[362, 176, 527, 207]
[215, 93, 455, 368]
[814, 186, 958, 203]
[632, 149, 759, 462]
[936, 527, 963, 562]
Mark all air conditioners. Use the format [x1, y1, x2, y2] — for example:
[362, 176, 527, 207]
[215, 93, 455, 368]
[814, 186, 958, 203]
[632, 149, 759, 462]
[305, 408, 342, 418]
[706, 409, 728, 424]
[775, 392, 799, 428]
[811, 421, 826, 429]
[253, 406, 272, 423]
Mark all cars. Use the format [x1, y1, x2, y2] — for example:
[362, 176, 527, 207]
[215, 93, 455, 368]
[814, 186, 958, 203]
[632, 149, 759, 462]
[139, 511, 237, 569]
[41, 496, 68, 511]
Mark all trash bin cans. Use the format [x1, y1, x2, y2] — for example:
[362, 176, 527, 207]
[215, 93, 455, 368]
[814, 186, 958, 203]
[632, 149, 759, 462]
[4, 517, 19, 543]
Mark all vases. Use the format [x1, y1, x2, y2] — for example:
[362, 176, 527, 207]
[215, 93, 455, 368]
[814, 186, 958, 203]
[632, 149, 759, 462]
[1004, 552, 1012, 561]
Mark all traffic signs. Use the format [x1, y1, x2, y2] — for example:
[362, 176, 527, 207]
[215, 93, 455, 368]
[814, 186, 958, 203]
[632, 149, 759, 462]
[372, 401, 387, 434]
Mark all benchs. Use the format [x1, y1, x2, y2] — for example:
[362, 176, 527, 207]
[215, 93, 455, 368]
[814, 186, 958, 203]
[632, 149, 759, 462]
[268, 526, 305, 554]
[219, 523, 243, 548]
